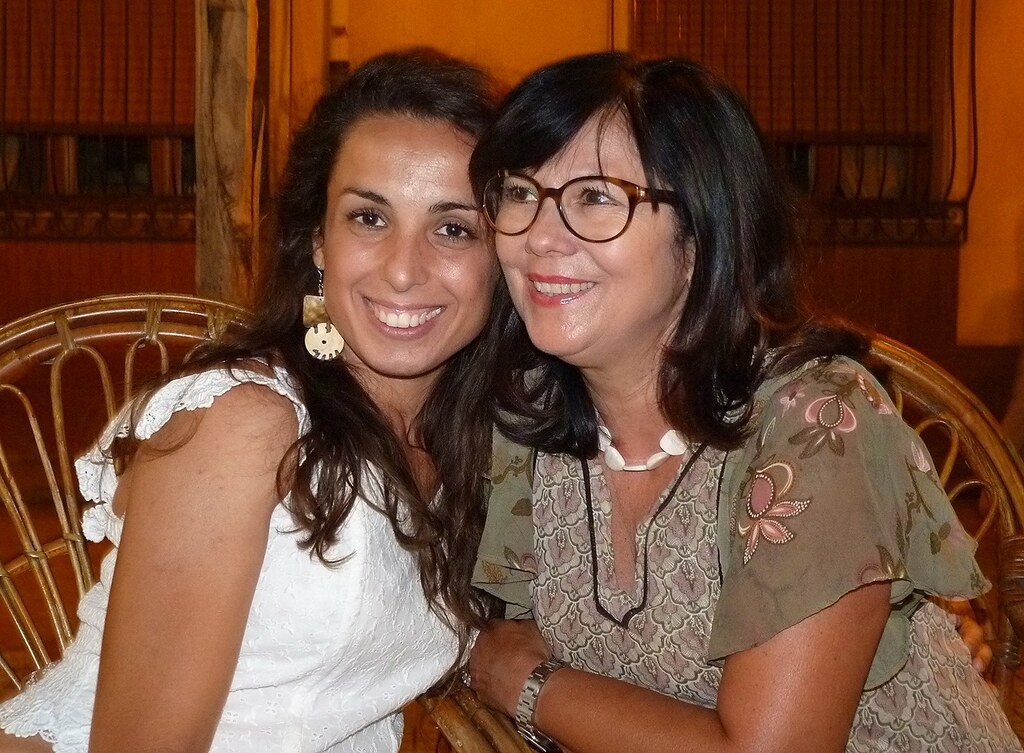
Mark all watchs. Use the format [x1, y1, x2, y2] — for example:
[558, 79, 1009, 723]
[515, 658, 572, 753]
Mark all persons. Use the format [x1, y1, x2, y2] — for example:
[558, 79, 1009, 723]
[458, 50, 1024, 753]
[0, 47, 993, 752]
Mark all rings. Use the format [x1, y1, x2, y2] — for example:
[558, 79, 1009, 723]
[462, 672, 471, 688]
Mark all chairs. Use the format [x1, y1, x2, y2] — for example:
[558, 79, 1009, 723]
[0, 290, 1024, 753]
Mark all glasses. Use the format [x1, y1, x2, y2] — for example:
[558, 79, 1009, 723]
[482, 173, 679, 244]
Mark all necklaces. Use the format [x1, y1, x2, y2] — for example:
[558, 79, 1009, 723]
[593, 403, 689, 471]
